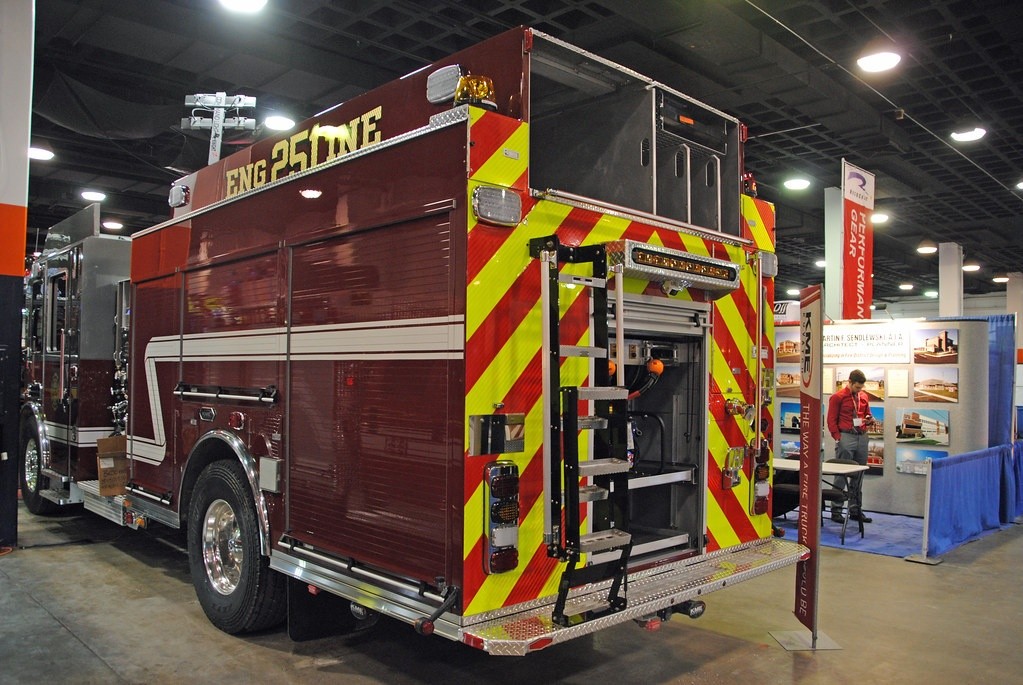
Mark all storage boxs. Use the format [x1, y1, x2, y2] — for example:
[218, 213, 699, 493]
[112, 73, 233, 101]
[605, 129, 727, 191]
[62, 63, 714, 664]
[96, 434, 132, 497]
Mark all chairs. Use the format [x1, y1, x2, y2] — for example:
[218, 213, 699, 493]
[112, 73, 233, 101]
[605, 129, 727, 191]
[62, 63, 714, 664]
[821, 458, 863, 538]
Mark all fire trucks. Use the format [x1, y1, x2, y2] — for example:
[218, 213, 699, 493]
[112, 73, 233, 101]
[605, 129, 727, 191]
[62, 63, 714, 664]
[14, 25, 812, 657]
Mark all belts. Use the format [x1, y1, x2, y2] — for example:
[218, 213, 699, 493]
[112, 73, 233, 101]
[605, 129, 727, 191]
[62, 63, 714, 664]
[838, 431, 866, 434]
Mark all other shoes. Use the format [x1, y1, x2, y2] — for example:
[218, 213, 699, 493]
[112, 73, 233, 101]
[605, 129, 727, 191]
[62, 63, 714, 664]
[850, 512, 872, 522]
[831, 514, 848, 523]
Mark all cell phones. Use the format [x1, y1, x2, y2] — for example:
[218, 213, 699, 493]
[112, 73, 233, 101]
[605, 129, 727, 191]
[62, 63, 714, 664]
[865, 416, 872, 420]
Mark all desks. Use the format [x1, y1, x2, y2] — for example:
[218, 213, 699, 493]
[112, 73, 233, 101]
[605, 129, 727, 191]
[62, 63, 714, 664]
[772, 457, 870, 545]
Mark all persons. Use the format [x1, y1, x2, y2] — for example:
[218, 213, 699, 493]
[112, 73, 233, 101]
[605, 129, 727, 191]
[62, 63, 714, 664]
[827, 370, 874, 523]
